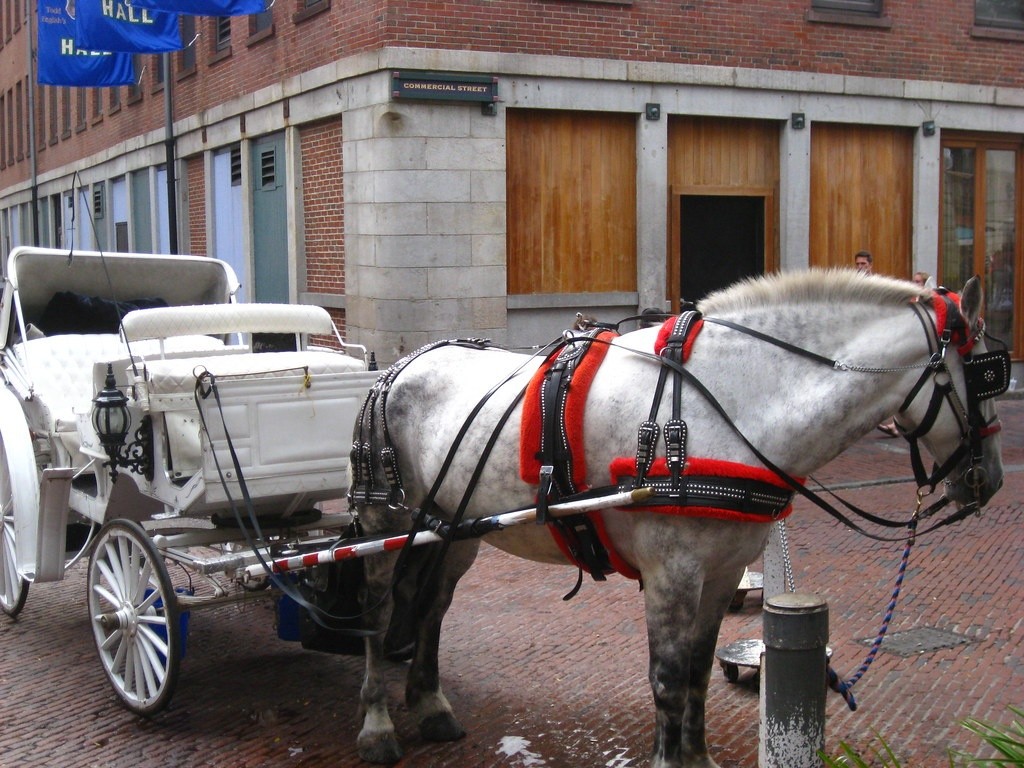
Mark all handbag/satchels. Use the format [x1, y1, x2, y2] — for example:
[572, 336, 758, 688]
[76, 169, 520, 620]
[42, 291, 168, 335]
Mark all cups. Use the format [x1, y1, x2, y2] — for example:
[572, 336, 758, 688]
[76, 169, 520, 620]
[1009, 379, 1017, 391]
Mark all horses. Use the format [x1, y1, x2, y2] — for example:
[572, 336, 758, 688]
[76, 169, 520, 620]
[346, 264, 1003, 768]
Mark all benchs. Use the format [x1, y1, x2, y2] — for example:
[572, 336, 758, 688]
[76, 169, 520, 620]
[118, 303, 369, 395]
[0, 246, 251, 475]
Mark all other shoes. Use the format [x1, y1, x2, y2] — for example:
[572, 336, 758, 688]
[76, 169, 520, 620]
[878, 424, 900, 437]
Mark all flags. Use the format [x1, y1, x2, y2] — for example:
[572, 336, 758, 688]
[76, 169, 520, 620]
[37, 0, 135, 87]
[132, 0, 268, 14]
[76, 0, 184, 53]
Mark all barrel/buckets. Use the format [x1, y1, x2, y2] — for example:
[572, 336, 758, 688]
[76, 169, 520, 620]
[269, 572, 302, 642]
[139, 556, 195, 665]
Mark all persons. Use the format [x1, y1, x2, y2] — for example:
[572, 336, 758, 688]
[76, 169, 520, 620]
[879, 272, 933, 436]
[855, 251, 873, 274]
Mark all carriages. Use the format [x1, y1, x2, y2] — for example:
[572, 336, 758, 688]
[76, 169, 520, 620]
[0, 245, 1011, 768]
